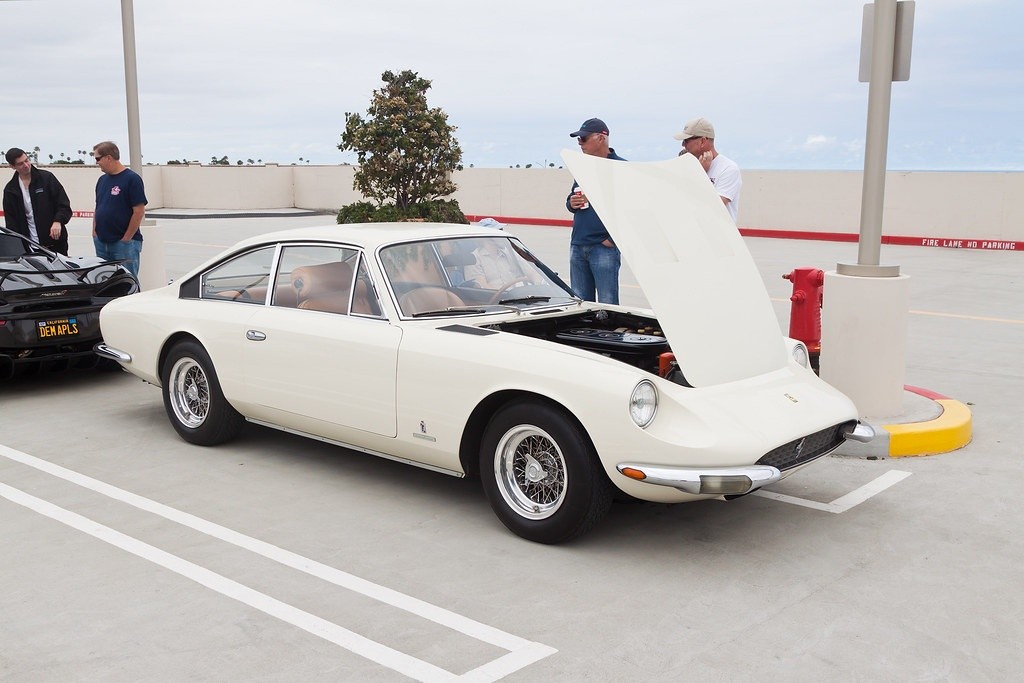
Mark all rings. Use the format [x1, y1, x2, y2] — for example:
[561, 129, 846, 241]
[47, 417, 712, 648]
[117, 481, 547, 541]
[702, 157, 707, 161]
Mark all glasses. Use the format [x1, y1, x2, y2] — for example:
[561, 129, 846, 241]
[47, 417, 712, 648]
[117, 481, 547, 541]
[683, 137, 709, 144]
[578, 133, 600, 141]
[95, 155, 107, 161]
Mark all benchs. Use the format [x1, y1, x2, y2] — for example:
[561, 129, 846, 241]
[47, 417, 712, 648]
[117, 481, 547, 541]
[217, 278, 375, 309]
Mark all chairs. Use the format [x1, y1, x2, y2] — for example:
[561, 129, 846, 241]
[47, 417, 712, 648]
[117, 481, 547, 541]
[398, 286, 465, 316]
[291, 261, 372, 315]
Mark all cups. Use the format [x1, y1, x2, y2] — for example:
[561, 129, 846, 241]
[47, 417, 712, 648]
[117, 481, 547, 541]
[574, 186, 589, 209]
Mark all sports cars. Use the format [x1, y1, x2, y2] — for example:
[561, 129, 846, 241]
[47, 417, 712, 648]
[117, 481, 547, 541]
[91, 150, 877, 545]
[0, 226, 143, 377]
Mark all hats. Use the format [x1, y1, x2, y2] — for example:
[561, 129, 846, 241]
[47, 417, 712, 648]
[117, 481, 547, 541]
[477, 218, 508, 230]
[570, 118, 609, 137]
[673, 117, 715, 140]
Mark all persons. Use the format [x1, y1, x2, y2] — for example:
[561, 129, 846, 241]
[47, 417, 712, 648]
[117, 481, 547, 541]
[2, 148, 73, 256]
[673, 117, 743, 224]
[463, 217, 516, 292]
[92, 141, 148, 278]
[565, 117, 628, 305]
